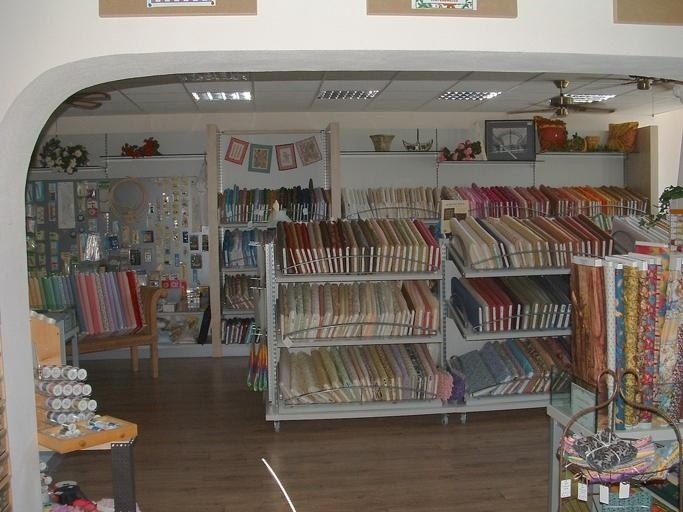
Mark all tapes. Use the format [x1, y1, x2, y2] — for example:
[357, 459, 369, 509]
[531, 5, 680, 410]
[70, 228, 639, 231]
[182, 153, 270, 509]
[162, 280, 178, 288]
[88, 208, 95, 215]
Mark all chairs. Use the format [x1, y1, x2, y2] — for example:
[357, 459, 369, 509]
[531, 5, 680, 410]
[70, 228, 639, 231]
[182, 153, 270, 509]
[66, 286, 168, 378]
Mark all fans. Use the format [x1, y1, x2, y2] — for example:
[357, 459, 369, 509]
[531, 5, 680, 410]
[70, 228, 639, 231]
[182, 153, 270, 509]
[506, 80, 616, 127]
[69, 92, 111, 111]
[592, 75, 683, 117]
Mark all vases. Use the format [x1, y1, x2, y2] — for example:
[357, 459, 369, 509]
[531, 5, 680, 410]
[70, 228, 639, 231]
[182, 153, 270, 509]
[368, 134, 394, 152]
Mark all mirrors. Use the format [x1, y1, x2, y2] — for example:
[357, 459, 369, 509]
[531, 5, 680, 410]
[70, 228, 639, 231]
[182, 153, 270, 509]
[109, 176, 148, 225]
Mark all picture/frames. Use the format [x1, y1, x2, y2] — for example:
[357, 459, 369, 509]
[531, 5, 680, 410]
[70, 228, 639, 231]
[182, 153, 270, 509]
[99, 0, 257, 16]
[484, 120, 536, 162]
[613, 0, 682, 28]
[366, 0, 518, 19]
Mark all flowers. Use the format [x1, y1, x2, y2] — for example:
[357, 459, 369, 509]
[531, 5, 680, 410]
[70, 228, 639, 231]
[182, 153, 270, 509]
[120, 135, 162, 159]
[437, 138, 481, 162]
[39, 138, 91, 175]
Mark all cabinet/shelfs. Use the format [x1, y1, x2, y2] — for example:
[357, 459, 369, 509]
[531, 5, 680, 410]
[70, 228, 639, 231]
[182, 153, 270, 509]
[444, 215, 671, 426]
[261, 227, 451, 432]
[206, 124, 340, 358]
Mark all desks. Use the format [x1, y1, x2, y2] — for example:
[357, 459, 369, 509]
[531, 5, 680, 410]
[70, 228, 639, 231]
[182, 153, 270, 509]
[546, 364, 683, 512]
[64, 326, 80, 370]
[37, 414, 138, 512]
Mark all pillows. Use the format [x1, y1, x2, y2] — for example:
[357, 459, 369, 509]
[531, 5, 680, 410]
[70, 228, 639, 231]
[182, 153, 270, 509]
[533, 115, 567, 152]
[607, 122, 638, 153]
